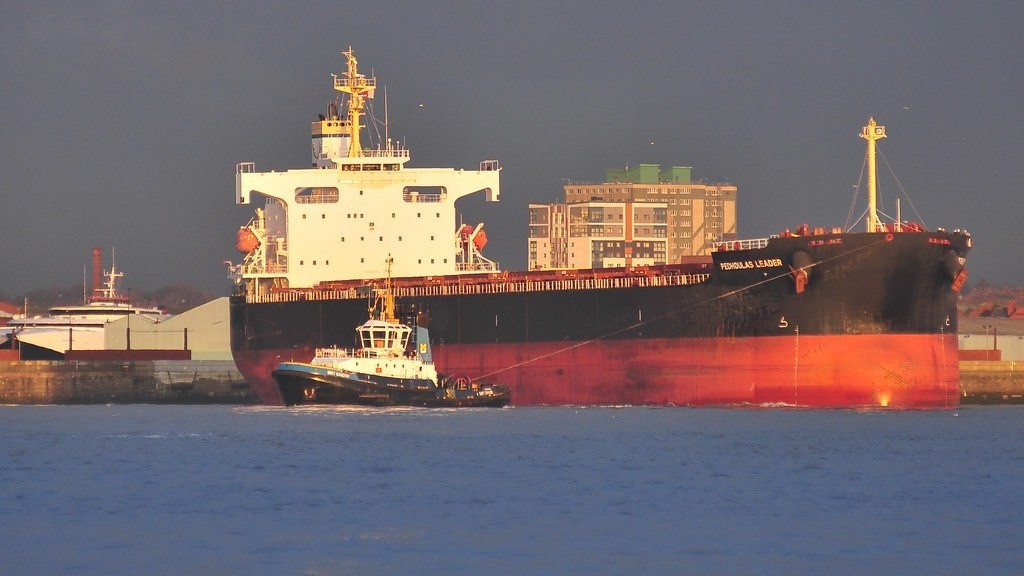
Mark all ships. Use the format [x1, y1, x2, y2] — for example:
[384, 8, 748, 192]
[224, 45, 973, 411]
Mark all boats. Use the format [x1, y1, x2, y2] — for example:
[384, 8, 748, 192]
[269, 252, 511, 410]
[461, 223, 488, 252]
[232, 227, 260, 255]
